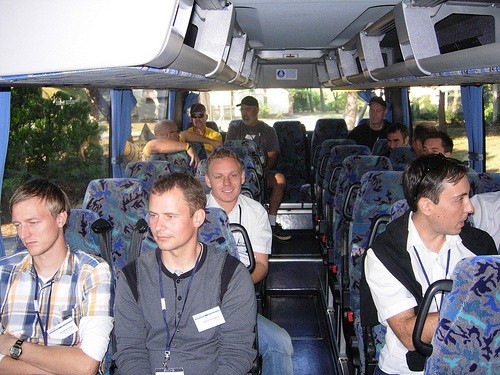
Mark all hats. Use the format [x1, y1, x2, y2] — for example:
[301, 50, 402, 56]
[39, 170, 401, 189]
[190, 103, 206, 113]
[367, 97, 386, 107]
[236, 96, 258, 108]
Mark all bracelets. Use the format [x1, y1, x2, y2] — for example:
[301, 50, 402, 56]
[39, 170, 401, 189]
[185, 144, 191, 152]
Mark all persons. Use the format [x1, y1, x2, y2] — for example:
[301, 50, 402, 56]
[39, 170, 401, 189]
[179, 103, 222, 158]
[0, 178, 113, 375]
[113, 173, 257, 375]
[377, 122, 410, 158]
[422, 131, 453, 158]
[410, 123, 437, 157]
[346, 96, 391, 152]
[144, 119, 200, 169]
[359, 154, 500, 375]
[224, 96, 291, 241]
[206, 147, 294, 375]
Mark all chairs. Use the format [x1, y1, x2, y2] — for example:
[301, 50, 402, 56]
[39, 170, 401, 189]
[11, 117, 500, 375]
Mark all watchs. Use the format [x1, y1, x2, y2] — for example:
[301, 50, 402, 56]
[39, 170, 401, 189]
[9, 338, 25, 360]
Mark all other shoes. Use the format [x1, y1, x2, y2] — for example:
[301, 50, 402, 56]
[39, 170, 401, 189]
[271, 222, 292, 241]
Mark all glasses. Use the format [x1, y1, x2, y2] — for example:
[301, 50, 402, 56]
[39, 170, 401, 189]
[412, 152, 446, 201]
[170, 129, 180, 134]
[191, 114, 204, 118]
